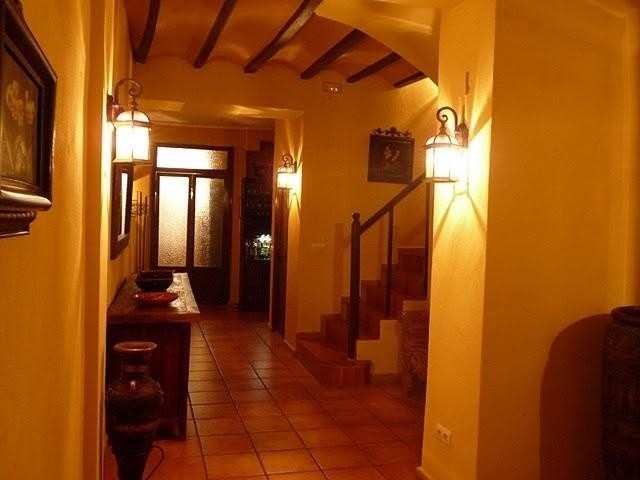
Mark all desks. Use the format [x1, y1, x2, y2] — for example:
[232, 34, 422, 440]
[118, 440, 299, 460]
[107, 273, 200, 441]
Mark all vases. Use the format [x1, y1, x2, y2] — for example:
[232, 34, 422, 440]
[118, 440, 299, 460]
[603, 306, 640, 480]
[105, 342, 165, 480]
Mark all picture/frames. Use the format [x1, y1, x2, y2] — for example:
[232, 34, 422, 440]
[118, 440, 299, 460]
[367, 126, 415, 185]
[0, 0, 58, 237]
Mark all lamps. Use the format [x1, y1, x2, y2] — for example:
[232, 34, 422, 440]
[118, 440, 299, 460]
[277, 154, 296, 190]
[424, 106, 469, 184]
[107, 78, 153, 167]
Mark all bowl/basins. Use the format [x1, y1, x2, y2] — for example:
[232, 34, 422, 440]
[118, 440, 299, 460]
[132, 292, 178, 308]
[134, 272, 174, 290]
